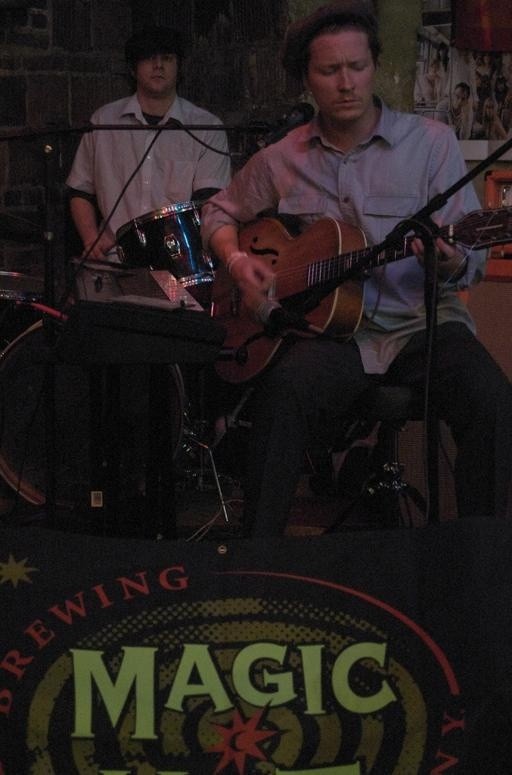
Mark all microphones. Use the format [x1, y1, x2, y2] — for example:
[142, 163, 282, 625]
[254, 299, 326, 338]
[246, 102, 315, 156]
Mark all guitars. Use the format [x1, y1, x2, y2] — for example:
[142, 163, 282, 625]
[206, 206, 512, 385]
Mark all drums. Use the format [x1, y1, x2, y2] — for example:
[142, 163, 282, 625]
[113, 200, 222, 288]
[0, 272, 45, 349]
[2, 315, 186, 511]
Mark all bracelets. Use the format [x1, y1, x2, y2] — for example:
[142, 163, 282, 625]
[223, 250, 246, 277]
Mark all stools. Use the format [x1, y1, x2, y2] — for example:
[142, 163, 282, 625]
[328, 380, 433, 530]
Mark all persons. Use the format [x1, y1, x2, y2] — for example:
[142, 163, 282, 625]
[414, 51, 511, 142]
[57, 25, 234, 541]
[199, 1, 512, 535]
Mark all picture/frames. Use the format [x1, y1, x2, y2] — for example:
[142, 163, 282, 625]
[482, 171, 511, 283]
